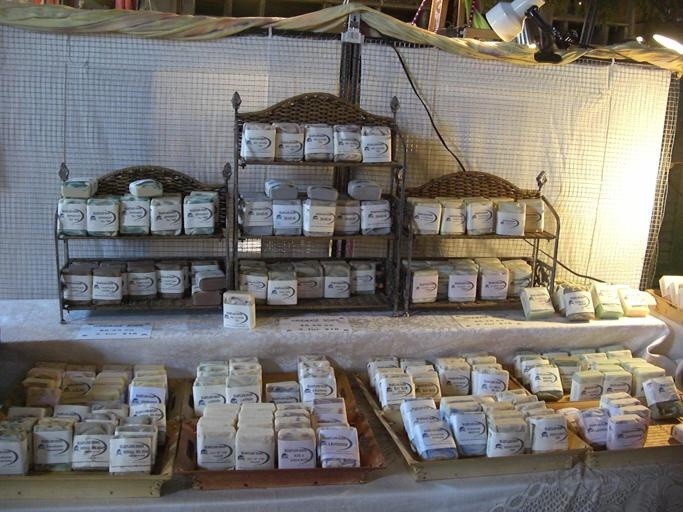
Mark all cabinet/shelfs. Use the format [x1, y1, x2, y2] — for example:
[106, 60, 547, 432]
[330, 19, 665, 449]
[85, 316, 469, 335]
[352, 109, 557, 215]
[53, 159, 231, 323]
[406, 172, 562, 314]
[230, 89, 405, 315]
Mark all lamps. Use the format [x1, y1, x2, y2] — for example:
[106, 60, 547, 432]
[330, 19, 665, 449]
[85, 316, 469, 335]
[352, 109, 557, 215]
[484, 0, 567, 63]
[652, 24, 682, 54]
[634, 33, 643, 46]
